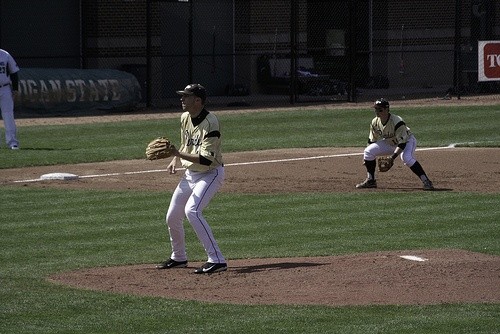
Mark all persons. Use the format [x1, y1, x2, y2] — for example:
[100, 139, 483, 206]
[146, 84, 228, 274]
[0, 49, 20, 149]
[356, 98, 434, 190]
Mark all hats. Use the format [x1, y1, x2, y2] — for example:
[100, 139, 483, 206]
[175, 84, 207, 97]
[371, 98, 389, 108]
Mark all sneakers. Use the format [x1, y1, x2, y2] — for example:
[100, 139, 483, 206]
[194, 262, 228, 274]
[424, 179, 433, 190]
[156, 259, 188, 269]
[355, 179, 377, 188]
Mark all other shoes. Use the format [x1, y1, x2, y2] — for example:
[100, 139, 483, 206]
[11, 145, 19, 150]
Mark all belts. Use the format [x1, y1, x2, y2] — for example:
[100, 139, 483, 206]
[0, 83, 9, 88]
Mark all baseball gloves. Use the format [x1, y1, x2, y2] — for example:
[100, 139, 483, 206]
[377, 156, 395, 172]
[145, 136, 176, 161]
